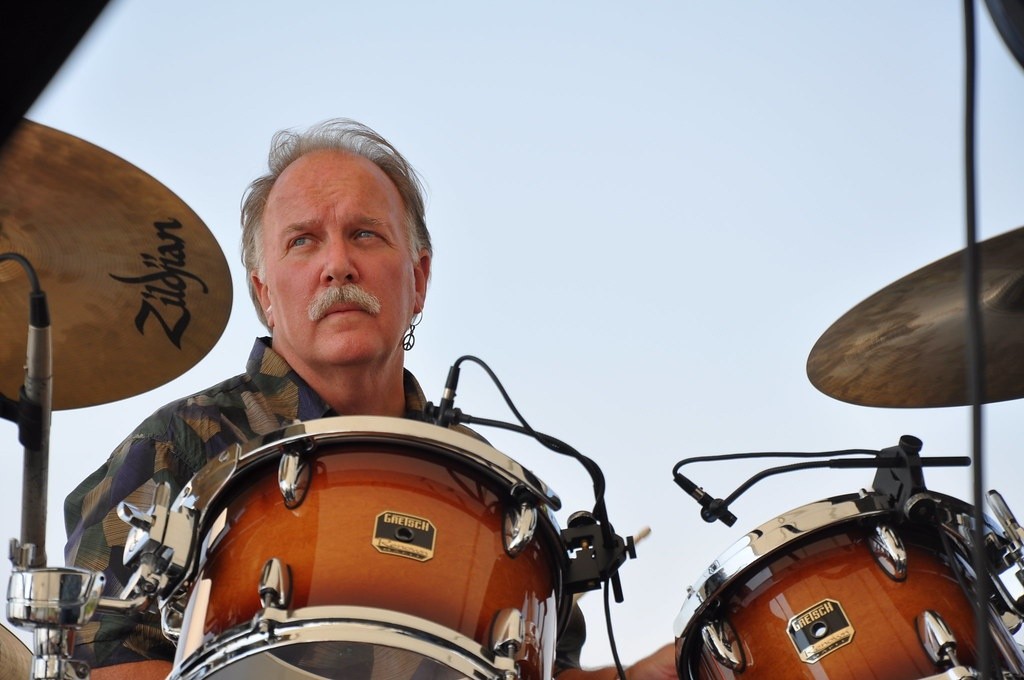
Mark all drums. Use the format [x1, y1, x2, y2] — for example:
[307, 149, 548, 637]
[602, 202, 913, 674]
[164, 415, 575, 680]
[672, 489, 1024, 680]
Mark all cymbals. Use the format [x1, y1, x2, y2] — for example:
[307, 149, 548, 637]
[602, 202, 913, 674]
[806, 226, 1024, 408]
[0, 116, 234, 411]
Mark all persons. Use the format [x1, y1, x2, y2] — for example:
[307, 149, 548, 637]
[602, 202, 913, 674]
[64, 122, 682, 680]
